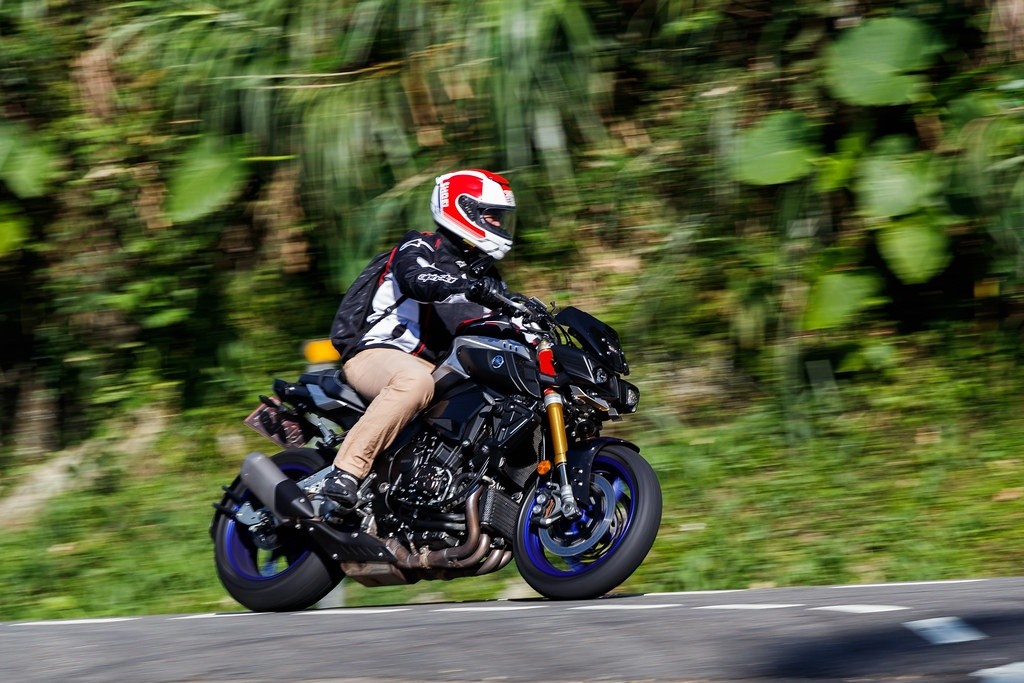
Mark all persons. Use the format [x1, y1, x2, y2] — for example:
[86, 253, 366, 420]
[323, 168, 517, 505]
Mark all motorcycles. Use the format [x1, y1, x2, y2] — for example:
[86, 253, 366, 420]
[208, 278, 663, 612]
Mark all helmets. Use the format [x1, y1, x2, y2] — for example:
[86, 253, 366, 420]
[431, 168, 517, 262]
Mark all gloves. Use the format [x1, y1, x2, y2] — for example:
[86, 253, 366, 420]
[465, 276, 506, 312]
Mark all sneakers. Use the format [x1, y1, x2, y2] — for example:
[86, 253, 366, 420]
[319, 469, 358, 509]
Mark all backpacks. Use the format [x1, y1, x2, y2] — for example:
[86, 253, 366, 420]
[329, 229, 438, 355]
